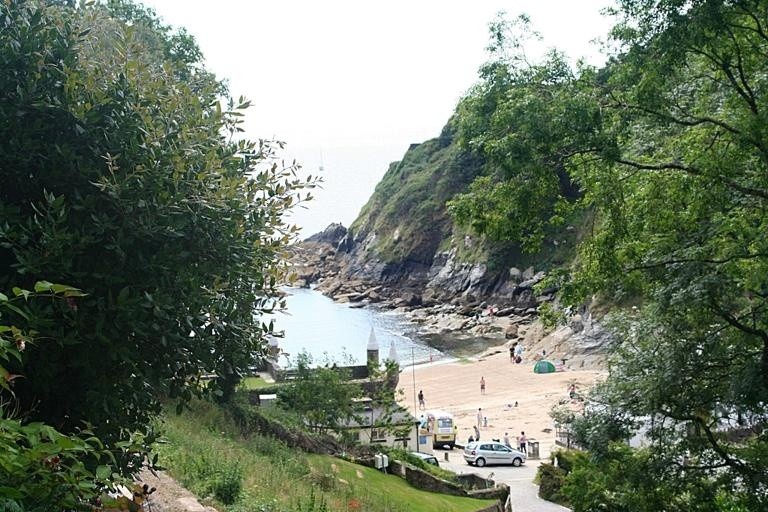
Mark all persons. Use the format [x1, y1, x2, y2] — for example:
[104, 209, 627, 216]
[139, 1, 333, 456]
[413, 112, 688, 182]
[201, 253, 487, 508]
[514, 401, 518, 406]
[420, 414, 427, 428]
[514, 354, 521, 363]
[519, 431, 527, 453]
[468, 435, 474, 443]
[515, 436, 520, 450]
[503, 431, 512, 448]
[479, 376, 486, 395]
[483, 417, 488, 427]
[454, 425, 458, 443]
[472, 425, 480, 441]
[509, 343, 515, 363]
[476, 407, 483, 429]
[504, 402, 512, 407]
[417, 390, 427, 410]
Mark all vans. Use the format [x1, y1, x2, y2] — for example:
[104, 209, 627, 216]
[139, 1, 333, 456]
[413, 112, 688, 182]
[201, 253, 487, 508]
[425, 408, 457, 450]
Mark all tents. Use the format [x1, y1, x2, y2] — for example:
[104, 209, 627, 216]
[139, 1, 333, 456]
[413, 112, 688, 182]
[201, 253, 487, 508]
[533, 361, 555, 373]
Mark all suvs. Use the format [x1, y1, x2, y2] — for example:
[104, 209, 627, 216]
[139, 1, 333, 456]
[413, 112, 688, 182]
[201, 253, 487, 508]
[464, 441, 527, 467]
